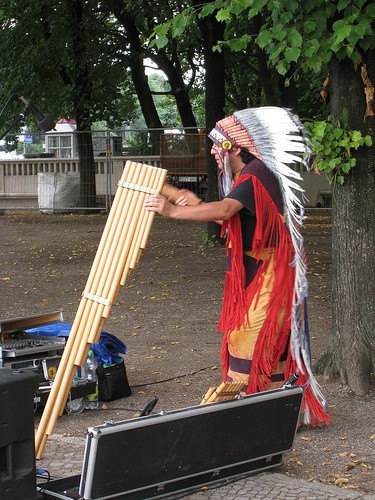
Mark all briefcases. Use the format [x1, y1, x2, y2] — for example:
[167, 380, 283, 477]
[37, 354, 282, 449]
[35, 385, 304, 500]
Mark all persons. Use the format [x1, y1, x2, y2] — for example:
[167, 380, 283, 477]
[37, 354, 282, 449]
[145, 107, 331, 431]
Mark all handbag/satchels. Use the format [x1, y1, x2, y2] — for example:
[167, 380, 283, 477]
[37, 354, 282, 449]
[94, 357, 132, 402]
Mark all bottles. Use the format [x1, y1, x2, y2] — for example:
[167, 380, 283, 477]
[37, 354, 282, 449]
[84, 350, 99, 410]
